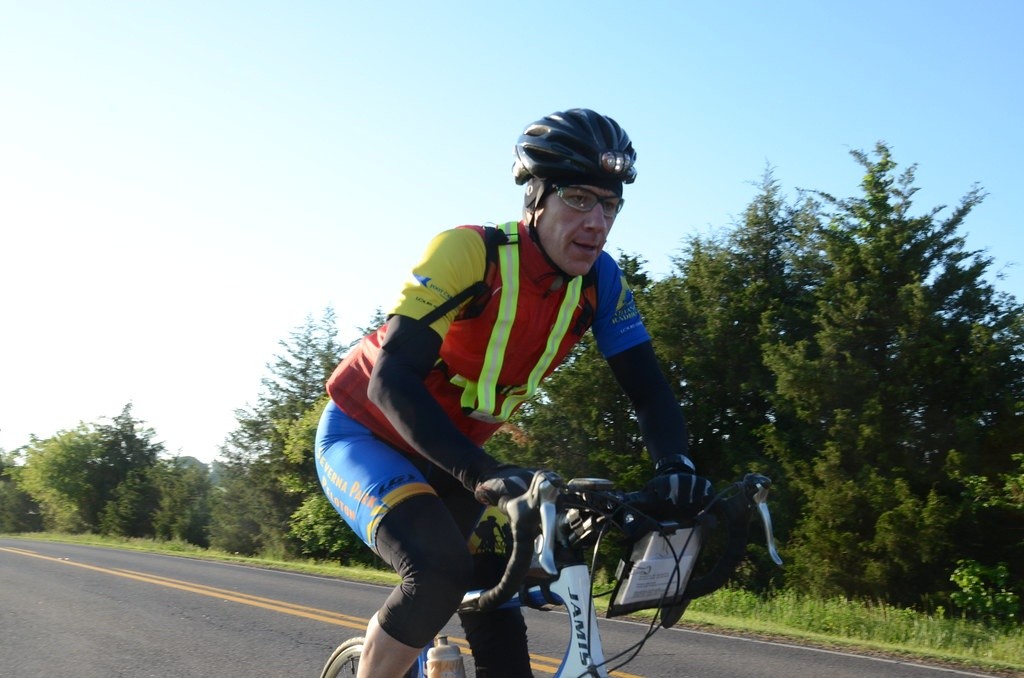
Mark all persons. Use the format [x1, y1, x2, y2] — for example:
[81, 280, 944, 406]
[315, 109, 718, 678]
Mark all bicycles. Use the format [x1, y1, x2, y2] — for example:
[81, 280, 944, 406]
[318, 465, 785, 678]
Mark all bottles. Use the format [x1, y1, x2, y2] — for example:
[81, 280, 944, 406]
[426, 635, 465, 678]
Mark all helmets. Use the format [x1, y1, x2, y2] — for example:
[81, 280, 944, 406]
[512, 107, 638, 186]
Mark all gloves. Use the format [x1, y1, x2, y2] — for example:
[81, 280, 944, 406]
[640, 453, 715, 516]
[462, 454, 538, 507]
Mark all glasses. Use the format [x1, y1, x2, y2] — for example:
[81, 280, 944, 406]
[552, 183, 625, 218]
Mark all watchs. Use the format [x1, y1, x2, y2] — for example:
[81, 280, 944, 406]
[655, 454, 697, 474]
